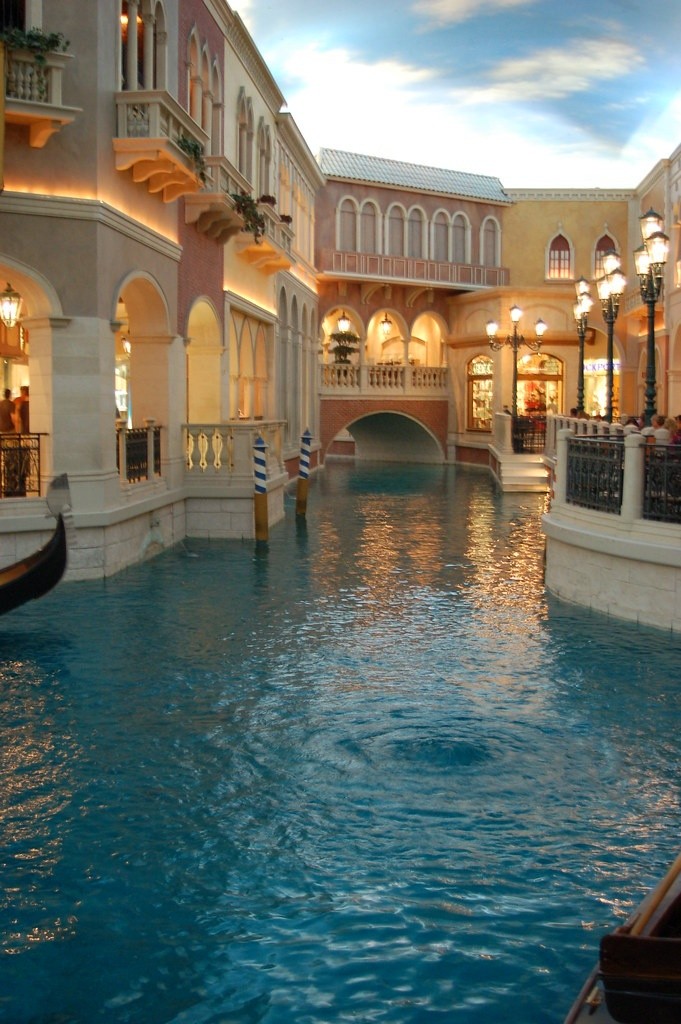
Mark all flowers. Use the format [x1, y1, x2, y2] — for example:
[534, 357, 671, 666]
[230, 186, 266, 245]
[257, 194, 277, 209]
[280, 214, 294, 225]
[180, 135, 208, 192]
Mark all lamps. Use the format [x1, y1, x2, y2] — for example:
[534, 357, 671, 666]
[338, 312, 350, 333]
[0, 283, 24, 330]
[381, 312, 392, 339]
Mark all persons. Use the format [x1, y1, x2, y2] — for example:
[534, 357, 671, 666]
[13, 386, 29, 433]
[570, 408, 681, 462]
[0, 388, 16, 434]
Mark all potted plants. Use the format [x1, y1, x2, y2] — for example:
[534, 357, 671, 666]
[3, 26, 70, 99]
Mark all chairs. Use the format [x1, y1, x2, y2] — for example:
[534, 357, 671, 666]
[512, 411, 545, 449]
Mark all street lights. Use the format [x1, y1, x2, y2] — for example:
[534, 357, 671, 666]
[573, 275, 593, 420]
[484, 303, 547, 453]
[631, 204, 672, 432]
[594, 246, 628, 424]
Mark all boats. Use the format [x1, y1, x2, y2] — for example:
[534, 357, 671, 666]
[0, 513, 68, 620]
